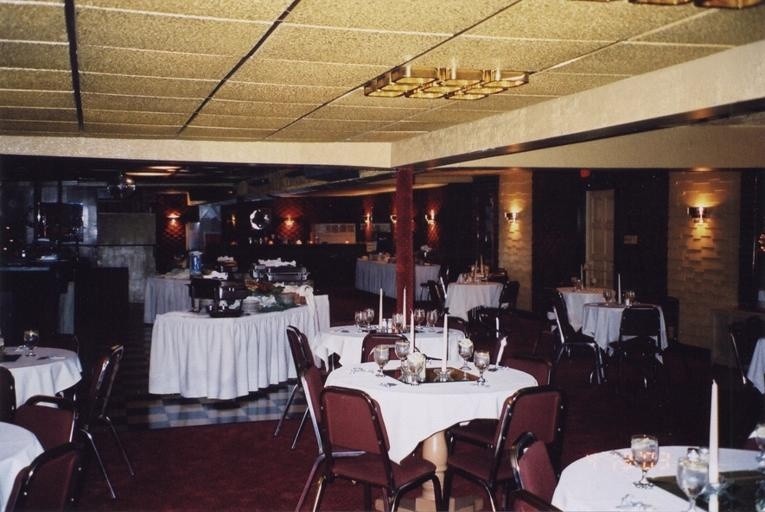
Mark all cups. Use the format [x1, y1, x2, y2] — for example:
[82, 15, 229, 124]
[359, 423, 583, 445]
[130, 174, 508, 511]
[427, 311, 437, 332]
[603, 289, 612, 304]
[570, 276, 578, 291]
[373, 346, 389, 376]
[474, 351, 489, 381]
[392, 314, 403, 333]
[414, 309, 424, 329]
[677, 448, 709, 512]
[458, 341, 473, 369]
[355, 313, 364, 332]
[756, 424, 765, 461]
[395, 340, 408, 379]
[24, 328, 40, 356]
[407, 354, 424, 384]
[625, 291, 636, 305]
[365, 309, 374, 329]
[631, 435, 659, 487]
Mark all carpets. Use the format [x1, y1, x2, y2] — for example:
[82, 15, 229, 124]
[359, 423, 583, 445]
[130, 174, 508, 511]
[74, 419, 369, 512]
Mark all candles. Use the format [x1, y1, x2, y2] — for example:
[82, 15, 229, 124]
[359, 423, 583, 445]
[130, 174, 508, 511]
[709, 379, 720, 511]
[410, 313, 415, 353]
[618, 273, 622, 305]
[379, 288, 383, 330]
[403, 289, 406, 329]
[441, 312, 448, 371]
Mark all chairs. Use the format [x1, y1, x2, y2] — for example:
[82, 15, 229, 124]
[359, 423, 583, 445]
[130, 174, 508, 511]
[505, 431, 564, 511]
[3, 444, 86, 512]
[444, 385, 568, 512]
[532, 288, 558, 356]
[78, 346, 135, 501]
[363, 333, 410, 363]
[311, 386, 443, 512]
[295, 362, 373, 512]
[447, 315, 468, 338]
[506, 351, 553, 385]
[10, 395, 79, 450]
[551, 303, 608, 386]
[468, 281, 521, 342]
[0, 366, 17, 423]
[609, 307, 667, 400]
[661, 296, 680, 352]
[274, 327, 328, 449]
[427, 279, 445, 309]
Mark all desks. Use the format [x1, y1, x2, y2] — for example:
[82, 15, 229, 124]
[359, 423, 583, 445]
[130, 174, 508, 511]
[712, 302, 765, 368]
[552, 446, 765, 512]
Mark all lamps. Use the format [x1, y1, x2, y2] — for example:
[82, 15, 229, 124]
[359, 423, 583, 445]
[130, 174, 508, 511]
[165, 211, 180, 224]
[364, 66, 529, 102]
[281, 213, 295, 226]
[505, 211, 521, 223]
[687, 206, 714, 224]
